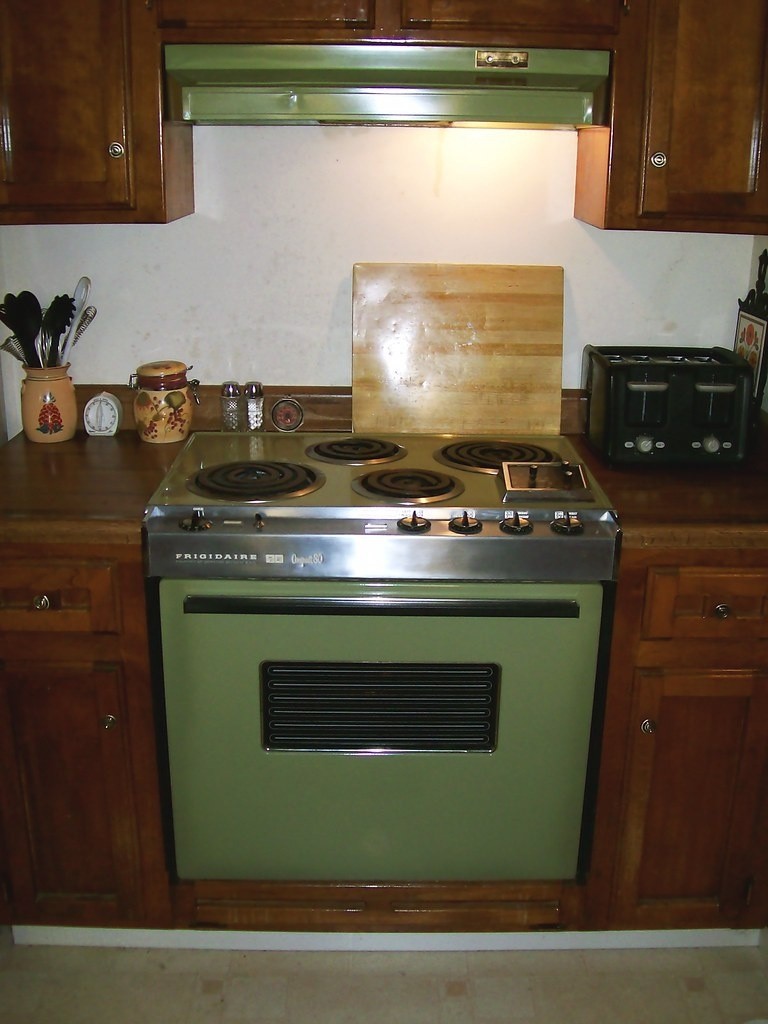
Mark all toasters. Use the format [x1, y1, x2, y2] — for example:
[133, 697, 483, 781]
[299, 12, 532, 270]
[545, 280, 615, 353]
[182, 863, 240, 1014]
[578, 345, 754, 473]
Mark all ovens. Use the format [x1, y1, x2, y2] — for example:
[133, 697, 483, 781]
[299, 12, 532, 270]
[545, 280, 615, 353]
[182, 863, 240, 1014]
[135, 582, 614, 881]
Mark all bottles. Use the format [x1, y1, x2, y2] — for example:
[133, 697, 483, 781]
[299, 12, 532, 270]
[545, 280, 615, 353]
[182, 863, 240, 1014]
[221, 380, 242, 432]
[244, 380, 265, 432]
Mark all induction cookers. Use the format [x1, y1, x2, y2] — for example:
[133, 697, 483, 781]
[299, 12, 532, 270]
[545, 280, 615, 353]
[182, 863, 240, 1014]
[141, 432, 624, 580]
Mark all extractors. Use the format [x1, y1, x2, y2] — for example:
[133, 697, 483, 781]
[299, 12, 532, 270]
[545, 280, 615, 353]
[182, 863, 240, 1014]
[159, 45, 611, 126]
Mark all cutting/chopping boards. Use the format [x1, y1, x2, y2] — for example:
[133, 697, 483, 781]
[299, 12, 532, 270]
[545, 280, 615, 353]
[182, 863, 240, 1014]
[353, 263, 566, 435]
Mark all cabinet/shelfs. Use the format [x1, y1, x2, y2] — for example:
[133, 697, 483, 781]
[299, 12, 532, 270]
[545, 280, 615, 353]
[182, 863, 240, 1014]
[0, 0, 194, 224]
[1, 544, 144, 928]
[574, 0, 768, 235]
[157, 0, 622, 47]
[608, 559, 768, 928]
